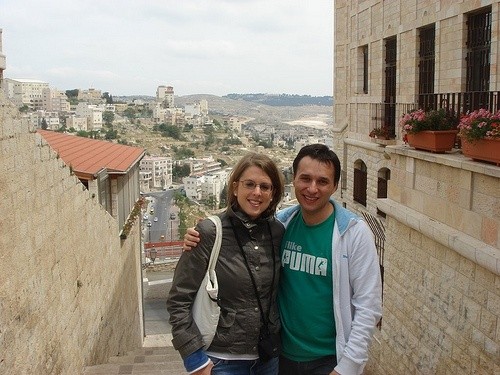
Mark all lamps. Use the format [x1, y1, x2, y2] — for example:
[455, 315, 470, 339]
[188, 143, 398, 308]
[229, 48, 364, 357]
[142, 245, 157, 270]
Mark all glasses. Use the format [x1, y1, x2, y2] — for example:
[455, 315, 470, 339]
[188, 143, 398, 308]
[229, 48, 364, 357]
[237, 179, 274, 193]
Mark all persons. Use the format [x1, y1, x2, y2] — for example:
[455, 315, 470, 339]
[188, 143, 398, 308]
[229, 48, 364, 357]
[165, 153, 286, 375]
[183, 144, 382, 375]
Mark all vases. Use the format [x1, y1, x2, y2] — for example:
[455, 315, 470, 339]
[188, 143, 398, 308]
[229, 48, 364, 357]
[407, 130, 458, 153]
[457, 134, 500, 167]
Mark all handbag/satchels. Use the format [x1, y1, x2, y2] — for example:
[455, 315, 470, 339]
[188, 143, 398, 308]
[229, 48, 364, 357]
[191, 214, 223, 351]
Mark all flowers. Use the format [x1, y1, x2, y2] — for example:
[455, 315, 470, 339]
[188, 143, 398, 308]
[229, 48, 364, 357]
[457, 108, 500, 145]
[369, 126, 396, 140]
[397, 108, 459, 144]
